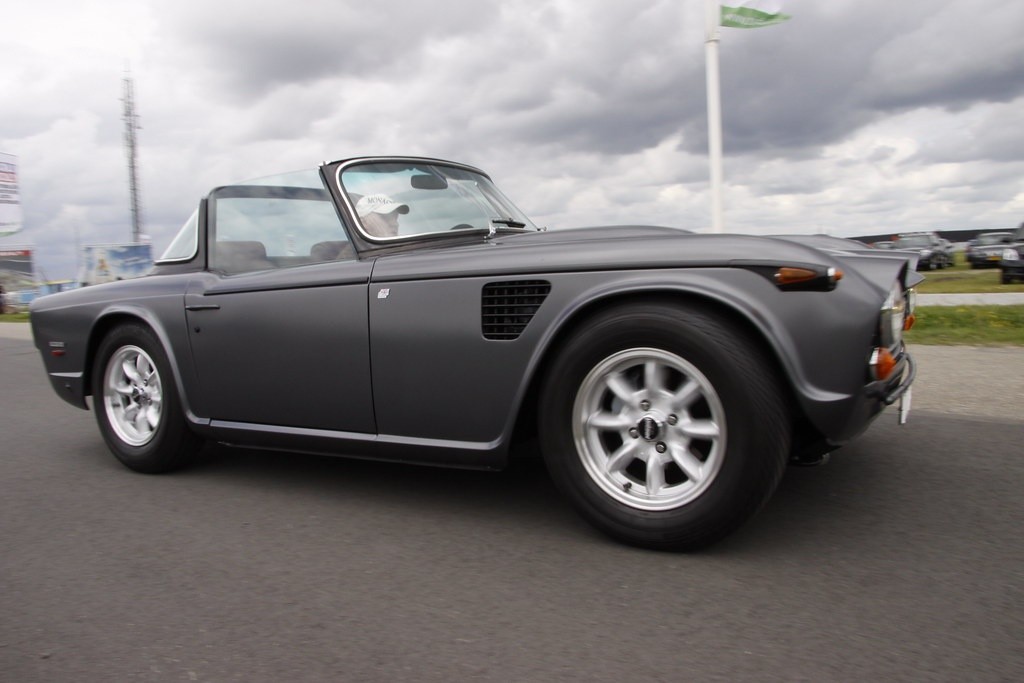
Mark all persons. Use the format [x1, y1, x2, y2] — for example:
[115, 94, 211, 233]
[330, 193, 412, 262]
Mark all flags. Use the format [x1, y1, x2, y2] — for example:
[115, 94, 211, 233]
[719, 1, 795, 29]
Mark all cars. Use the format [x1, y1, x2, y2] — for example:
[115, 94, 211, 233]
[26, 155, 925, 553]
[963, 231, 1015, 266]
[871, 231, 956, 269]
[998, 220, 1024, 282]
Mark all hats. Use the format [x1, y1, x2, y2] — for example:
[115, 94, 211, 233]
[355, 194, 409, 218]
